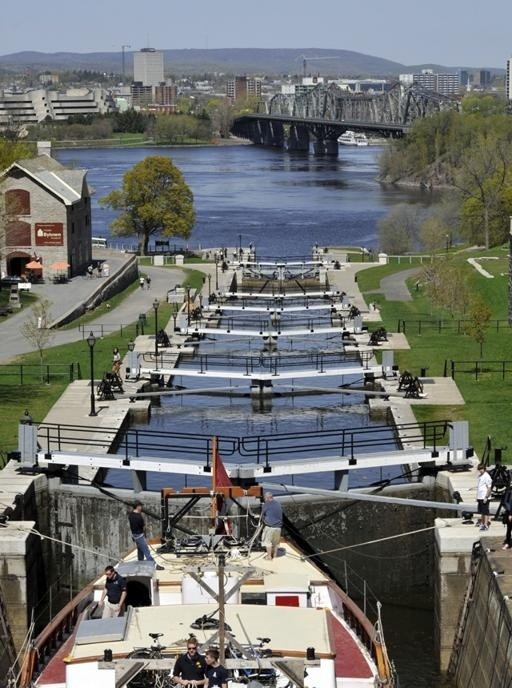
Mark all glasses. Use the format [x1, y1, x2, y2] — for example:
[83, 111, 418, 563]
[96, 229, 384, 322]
[188, 647, 196, 650]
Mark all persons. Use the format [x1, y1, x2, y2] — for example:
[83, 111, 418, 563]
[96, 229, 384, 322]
[97, 564, 130, 620]
[96, 261, 104, 278]
[212, 244, 228, 261]
[109, 346, 122, 385]
[129, 500, 166, 571]
[203, 650, 229, 688]
[86, 264, 93, 279]
[170, 635, 206, 688]
[258, 491, 283, 559]
[476, 463, 494, 531]
[146, 275, 151, 289]
[140, 276, 145, 290]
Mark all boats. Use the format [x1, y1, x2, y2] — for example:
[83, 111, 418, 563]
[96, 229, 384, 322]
[4, 432, 401, 688]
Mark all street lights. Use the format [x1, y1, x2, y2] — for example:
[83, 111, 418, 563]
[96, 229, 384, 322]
[86, 330, 98, 416]
[153, 298, 160, 356]
[186, 283, 191, 327]
